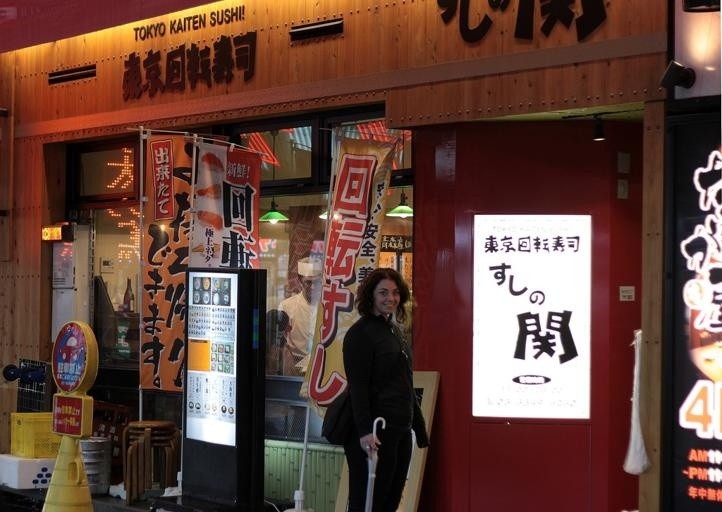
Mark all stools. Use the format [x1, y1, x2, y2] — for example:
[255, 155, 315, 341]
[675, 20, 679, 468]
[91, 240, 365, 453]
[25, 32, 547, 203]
[119, 419, 182, 506]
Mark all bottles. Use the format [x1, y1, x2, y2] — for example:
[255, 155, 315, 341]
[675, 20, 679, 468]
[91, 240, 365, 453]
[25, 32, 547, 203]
[123, 279, 134, 312]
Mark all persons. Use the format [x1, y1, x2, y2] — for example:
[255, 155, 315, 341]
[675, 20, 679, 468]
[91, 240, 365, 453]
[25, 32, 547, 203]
[343, 268, 429, 512]
[265, 309, 301, 418]
[278, 255, 323, 379]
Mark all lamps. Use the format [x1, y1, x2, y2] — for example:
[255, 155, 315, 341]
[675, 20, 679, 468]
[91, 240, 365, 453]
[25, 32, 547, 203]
[658, 58, 694, 89]
[259, 128, 289, 224]
[41, 221, 72, 241]
[386, 129, 414, 219]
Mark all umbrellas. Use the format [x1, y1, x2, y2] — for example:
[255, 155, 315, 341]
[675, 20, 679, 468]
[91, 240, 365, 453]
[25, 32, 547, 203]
[366, 414, 387, 512]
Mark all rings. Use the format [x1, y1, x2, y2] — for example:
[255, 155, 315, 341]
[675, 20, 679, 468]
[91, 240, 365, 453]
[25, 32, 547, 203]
[366, 445, 371, 450]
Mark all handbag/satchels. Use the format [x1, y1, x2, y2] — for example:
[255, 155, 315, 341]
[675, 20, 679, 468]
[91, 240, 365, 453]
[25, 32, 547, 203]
[320, 391, 361, 447]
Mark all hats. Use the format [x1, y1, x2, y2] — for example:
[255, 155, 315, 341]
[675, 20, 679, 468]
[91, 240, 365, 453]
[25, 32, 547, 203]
[296, 255, 324, 278]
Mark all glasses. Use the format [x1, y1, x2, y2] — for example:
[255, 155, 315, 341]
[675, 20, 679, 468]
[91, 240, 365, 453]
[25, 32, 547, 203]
[299, 278, 323, 288]
